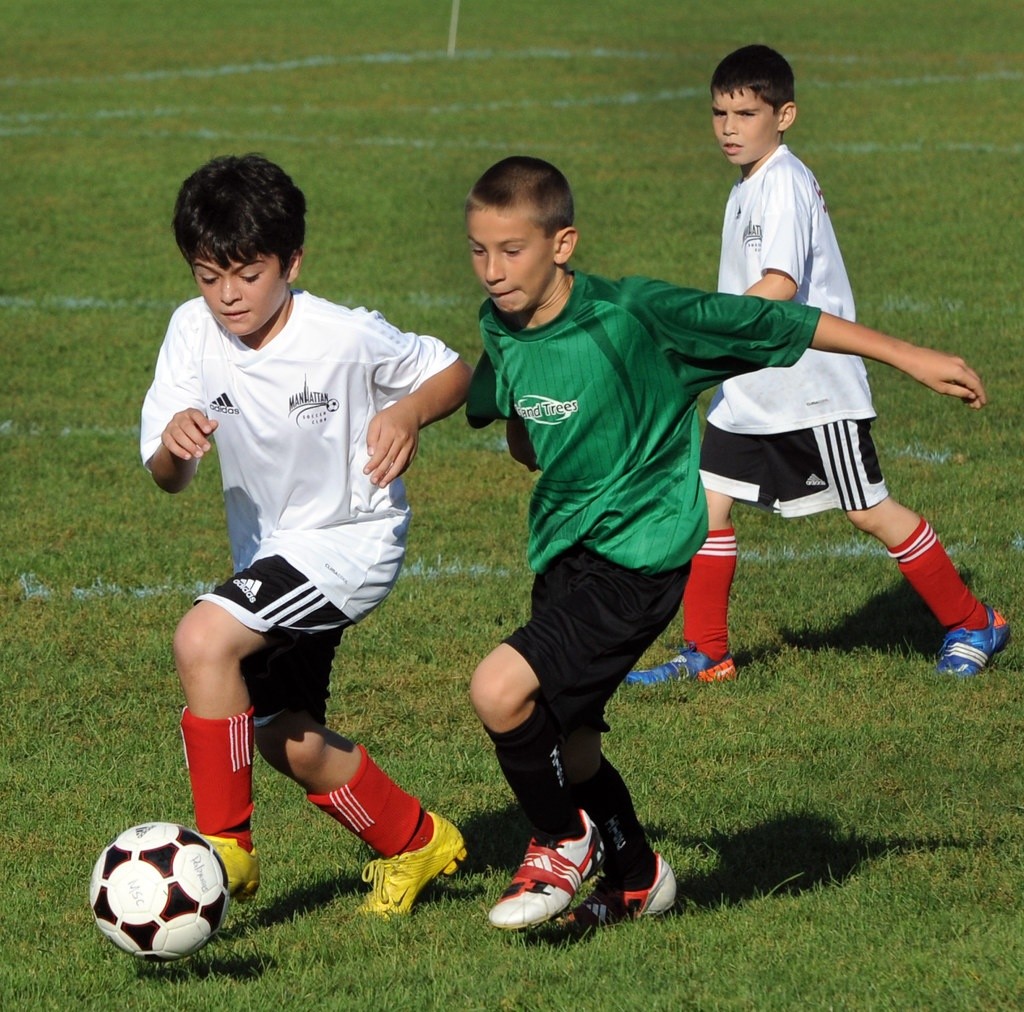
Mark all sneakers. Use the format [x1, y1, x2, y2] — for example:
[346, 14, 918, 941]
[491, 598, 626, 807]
[487, 809, 604, 930]
[357, 812, 467, 922]
[934, 604, 1011, 679]
[625, 650, 738, 685]
[197, 834, 260, 903]
[557, 852, 676, 933]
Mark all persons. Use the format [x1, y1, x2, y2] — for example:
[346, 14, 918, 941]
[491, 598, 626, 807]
[138, 148, 473, 922]
[462, 156, 991, 939]
[619, 43, 1013, 689]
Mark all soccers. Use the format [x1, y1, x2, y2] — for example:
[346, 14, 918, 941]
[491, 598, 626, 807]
[89, 821, 230, 963]
